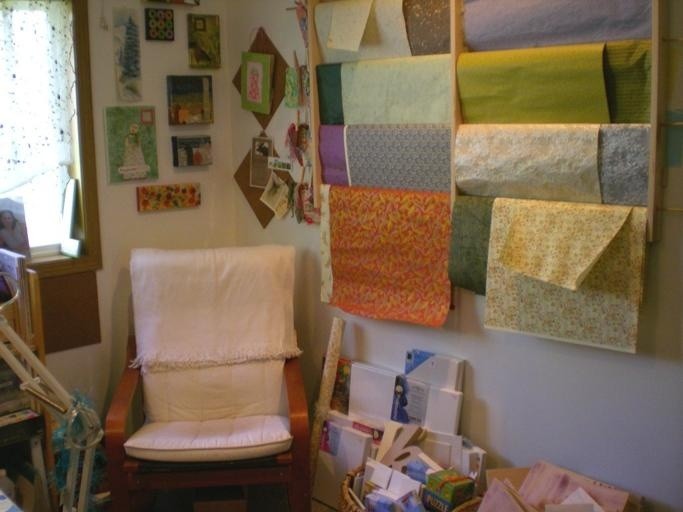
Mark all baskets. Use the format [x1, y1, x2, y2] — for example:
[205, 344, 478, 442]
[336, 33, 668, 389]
[339, 465, 483, 512]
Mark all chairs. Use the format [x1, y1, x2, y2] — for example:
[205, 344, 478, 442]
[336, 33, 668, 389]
[99, 242, 315, 510]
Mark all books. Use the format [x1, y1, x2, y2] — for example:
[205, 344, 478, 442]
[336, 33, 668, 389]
[318, 348, 488, 511]
[480, 461, 632, 512]
